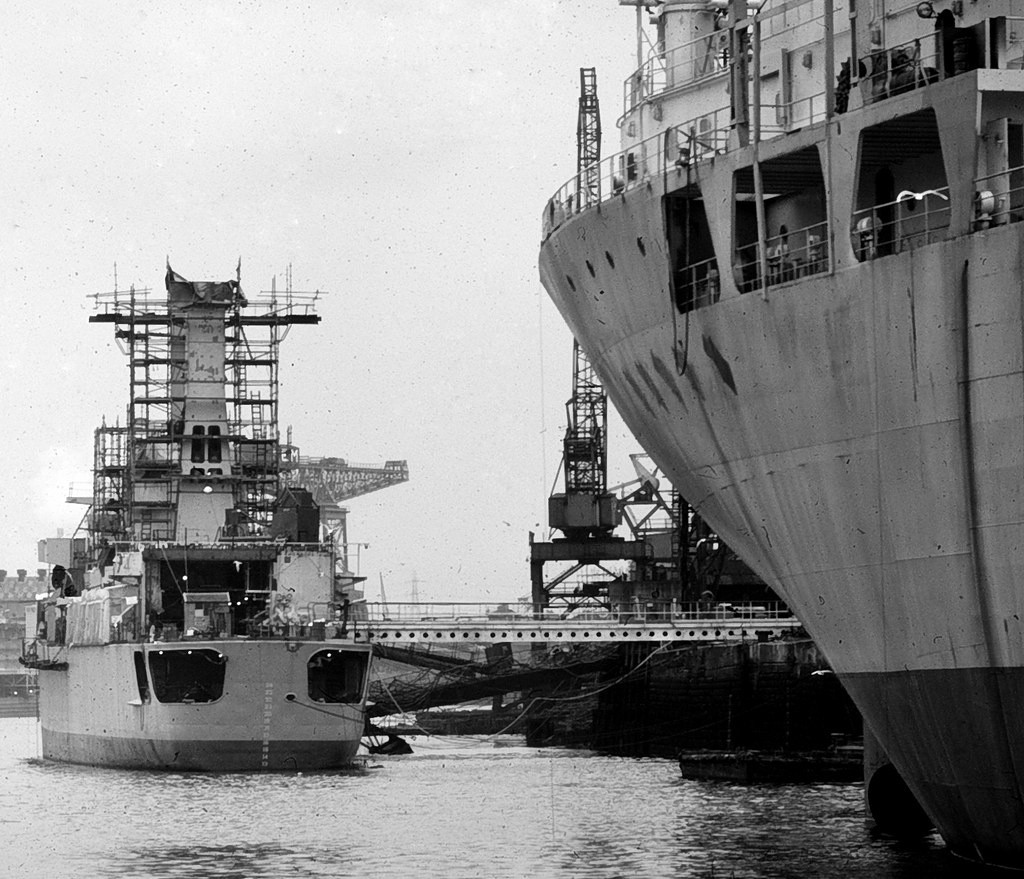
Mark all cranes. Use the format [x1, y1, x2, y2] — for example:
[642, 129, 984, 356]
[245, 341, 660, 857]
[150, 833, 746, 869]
[528, 68, 663, 620]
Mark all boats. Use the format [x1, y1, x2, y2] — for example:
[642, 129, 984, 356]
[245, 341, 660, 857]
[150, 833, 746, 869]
[675, 747, 825, 786]
[366, 732, 413, 756]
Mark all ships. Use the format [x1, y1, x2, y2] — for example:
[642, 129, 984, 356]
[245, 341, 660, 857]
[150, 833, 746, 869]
[14, 252, 378, 776]
[533, 0, 1024, 873]
